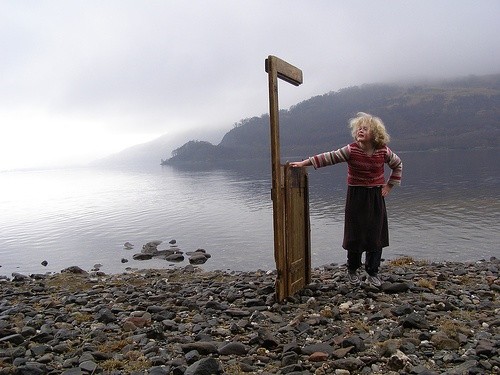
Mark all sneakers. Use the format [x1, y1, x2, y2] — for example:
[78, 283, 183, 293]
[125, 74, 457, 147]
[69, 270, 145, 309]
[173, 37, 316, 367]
[348, 273, 360, 284]
[367, 275, 381, 286]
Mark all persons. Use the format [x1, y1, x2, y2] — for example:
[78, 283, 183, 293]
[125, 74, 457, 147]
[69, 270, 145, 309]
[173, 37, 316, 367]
[288, 111, 403, 287]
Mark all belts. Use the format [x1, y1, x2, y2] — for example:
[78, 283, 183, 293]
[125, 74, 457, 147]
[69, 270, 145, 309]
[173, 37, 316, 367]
[360, 186, 381, 189]
